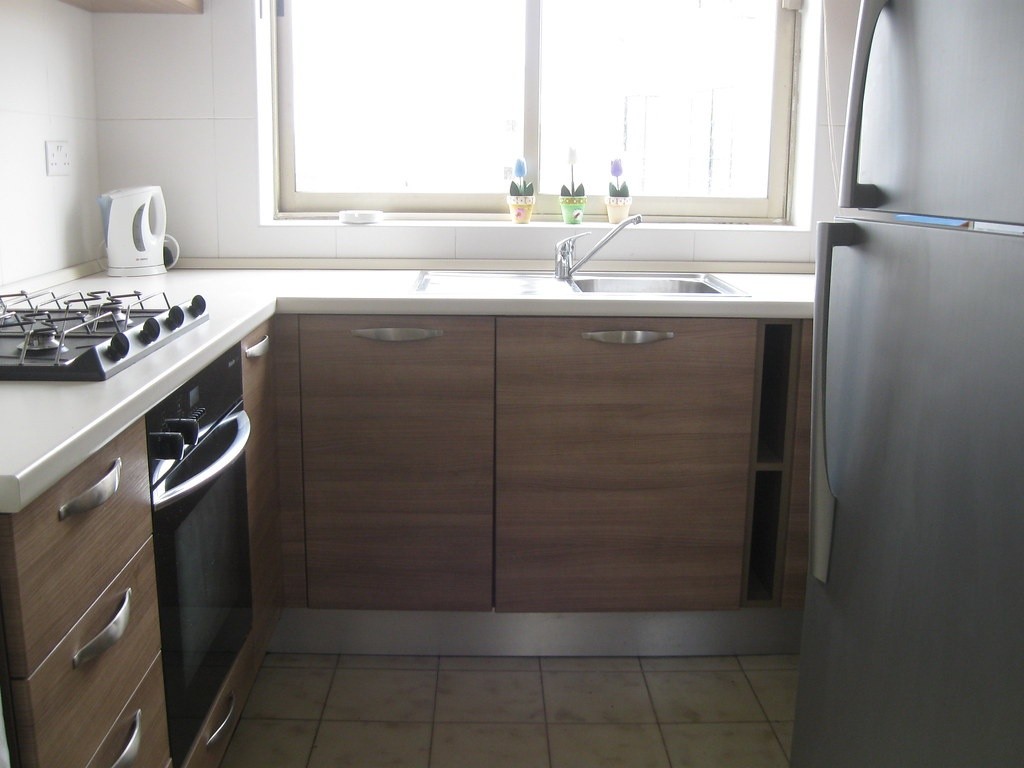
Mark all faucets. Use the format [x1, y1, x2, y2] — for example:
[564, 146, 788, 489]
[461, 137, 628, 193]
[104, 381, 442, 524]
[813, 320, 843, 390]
[555, 214, 642, 280]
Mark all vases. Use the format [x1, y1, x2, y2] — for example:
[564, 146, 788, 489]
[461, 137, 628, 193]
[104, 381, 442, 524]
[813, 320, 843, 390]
[605, 197, 633, 224]
[507, 196, 535, 224]
[559, 196, 587, 224]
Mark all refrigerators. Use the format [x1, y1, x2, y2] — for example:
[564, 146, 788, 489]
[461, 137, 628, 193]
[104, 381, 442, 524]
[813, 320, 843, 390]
[792, 0, 1024, 768]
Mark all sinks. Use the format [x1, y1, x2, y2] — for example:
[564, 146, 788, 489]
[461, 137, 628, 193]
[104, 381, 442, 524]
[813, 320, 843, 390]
[564, 272, 752, 298]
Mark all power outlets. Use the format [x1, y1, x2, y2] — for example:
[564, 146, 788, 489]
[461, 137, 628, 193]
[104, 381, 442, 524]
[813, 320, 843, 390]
[43, 140, 74, 177]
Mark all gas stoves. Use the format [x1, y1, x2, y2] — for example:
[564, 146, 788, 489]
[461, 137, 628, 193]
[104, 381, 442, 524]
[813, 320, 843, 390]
[0, 290, 210, 381]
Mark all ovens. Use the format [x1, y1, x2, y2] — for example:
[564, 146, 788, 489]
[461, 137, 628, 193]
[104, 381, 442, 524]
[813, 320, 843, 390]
[146, 342, 251, 768]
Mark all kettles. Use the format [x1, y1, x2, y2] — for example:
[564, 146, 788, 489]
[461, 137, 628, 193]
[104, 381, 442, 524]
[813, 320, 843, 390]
[94, 185, 169, 278]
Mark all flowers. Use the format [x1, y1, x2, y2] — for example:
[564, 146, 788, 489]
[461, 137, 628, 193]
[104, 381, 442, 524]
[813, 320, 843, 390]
[609, 158, 630, 197]
[510, 159, 534, 196]
[561, 148, 584, 197]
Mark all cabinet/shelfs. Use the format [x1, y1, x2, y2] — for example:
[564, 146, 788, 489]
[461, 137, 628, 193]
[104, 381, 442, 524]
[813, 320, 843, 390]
[0, 414, 182, 767]
[279, 316, 759, 656]
[241, 316, 277, 644]
